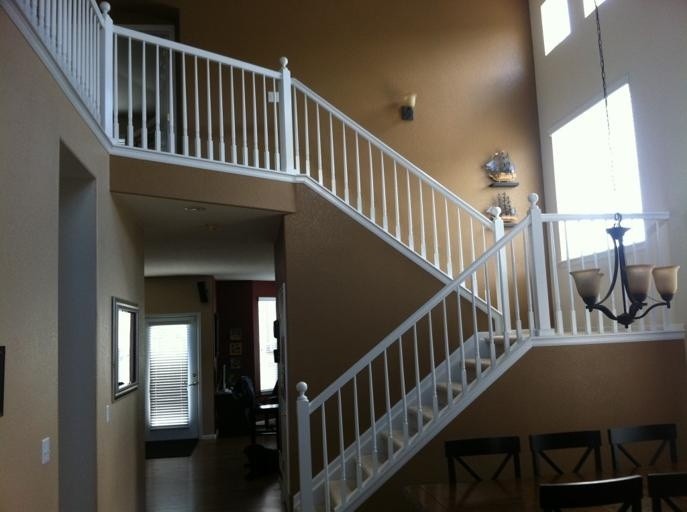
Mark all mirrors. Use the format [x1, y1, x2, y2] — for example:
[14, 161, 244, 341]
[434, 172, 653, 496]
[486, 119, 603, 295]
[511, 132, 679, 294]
[111, 297, 141, 403]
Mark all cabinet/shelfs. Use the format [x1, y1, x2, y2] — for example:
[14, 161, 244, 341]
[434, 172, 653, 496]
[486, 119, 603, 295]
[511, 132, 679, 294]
[488, 182, 520, 228]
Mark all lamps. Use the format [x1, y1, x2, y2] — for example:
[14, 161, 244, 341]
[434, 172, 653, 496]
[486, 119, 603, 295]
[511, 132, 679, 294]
[567, 1, 679, 327]
[399, 92, 416, 121]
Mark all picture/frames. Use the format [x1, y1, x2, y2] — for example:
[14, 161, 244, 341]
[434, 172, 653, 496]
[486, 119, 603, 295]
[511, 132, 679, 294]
[229, 325, 244, 387]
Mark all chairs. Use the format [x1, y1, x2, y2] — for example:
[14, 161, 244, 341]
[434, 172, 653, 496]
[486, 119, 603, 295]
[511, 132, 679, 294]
[402, 421, 686, 512]
[239, 376, 279, 447]
